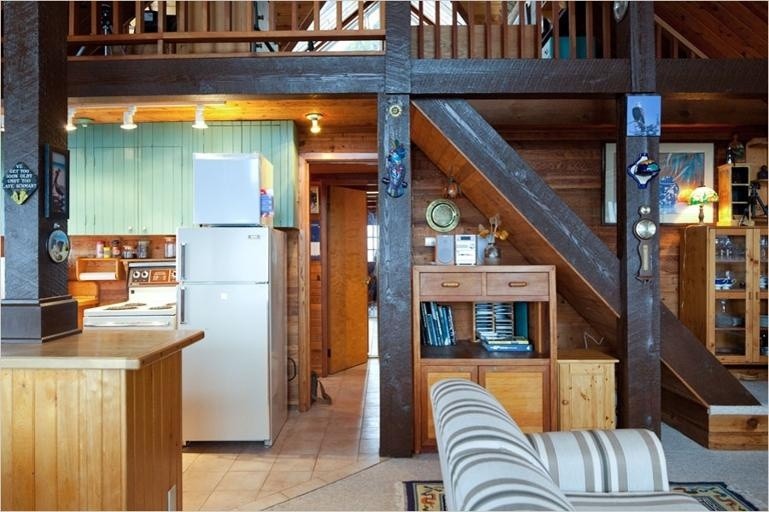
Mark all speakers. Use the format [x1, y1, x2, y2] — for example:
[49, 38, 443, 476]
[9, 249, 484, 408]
[433, 235, 454, 265]
[476, 235, 495, 265]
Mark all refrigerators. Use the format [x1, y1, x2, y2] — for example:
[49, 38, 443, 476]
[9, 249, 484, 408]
[172, 225, 289, 451]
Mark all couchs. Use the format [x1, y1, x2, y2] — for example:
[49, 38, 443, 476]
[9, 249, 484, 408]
[428, 377, 710, 510]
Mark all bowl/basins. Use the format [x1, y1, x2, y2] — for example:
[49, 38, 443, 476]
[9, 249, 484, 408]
[760, 314, 768, 327]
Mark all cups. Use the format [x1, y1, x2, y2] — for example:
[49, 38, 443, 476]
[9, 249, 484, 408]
[715, 278, 728, 289]
[762, 346, 768, 356]
[723, 278, 736, 289]
[760, 276, 767, 288]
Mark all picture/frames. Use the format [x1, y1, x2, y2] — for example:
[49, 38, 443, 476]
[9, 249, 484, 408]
[600, 139, 717, 226]
[43, 145, 69, 221]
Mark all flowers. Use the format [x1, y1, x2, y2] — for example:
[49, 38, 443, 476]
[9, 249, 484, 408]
[476, 214, 510, 241]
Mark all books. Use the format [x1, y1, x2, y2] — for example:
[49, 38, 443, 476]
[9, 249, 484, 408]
[430, 233, 497, 261]
[478, 332, 534, 353]
[421, 301, 456, 346]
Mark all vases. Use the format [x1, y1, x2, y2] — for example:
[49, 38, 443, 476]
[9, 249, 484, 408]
[483, 242, 501, 264]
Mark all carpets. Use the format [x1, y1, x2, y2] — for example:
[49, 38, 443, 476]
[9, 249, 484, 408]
[400, 479, 762, 512]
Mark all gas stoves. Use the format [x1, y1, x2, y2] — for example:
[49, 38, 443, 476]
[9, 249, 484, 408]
[82, 268, 175, 328]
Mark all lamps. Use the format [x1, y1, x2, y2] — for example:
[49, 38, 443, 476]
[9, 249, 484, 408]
[687, 185, 720, 224]
[192, 104, 208, 129]
[119, 104, 137, 129]
[64, 111, 78, 131]
[306, 112, 323, 133]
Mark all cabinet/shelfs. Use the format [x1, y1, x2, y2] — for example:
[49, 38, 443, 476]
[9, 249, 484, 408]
[66, 119, 300, 235]
[411, 265, 554, 452]
[719, 162, 755, 227]
[677, 223, 767, 384]
[746, 136, 768, 225]
[550, 347, 619, 432]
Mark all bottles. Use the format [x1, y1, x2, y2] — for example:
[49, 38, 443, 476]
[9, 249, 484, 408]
[715, 238, 734, 261]
[122, 245, 133, 258]
[163, 237, 176, 258]
[716, 300, 733, 328]
[760, 236, 768, 261]
[96, 240, 121, 258]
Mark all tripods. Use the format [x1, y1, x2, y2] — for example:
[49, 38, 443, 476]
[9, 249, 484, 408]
[739, 188, 768, 229]
[76, 15, 126, 57]
